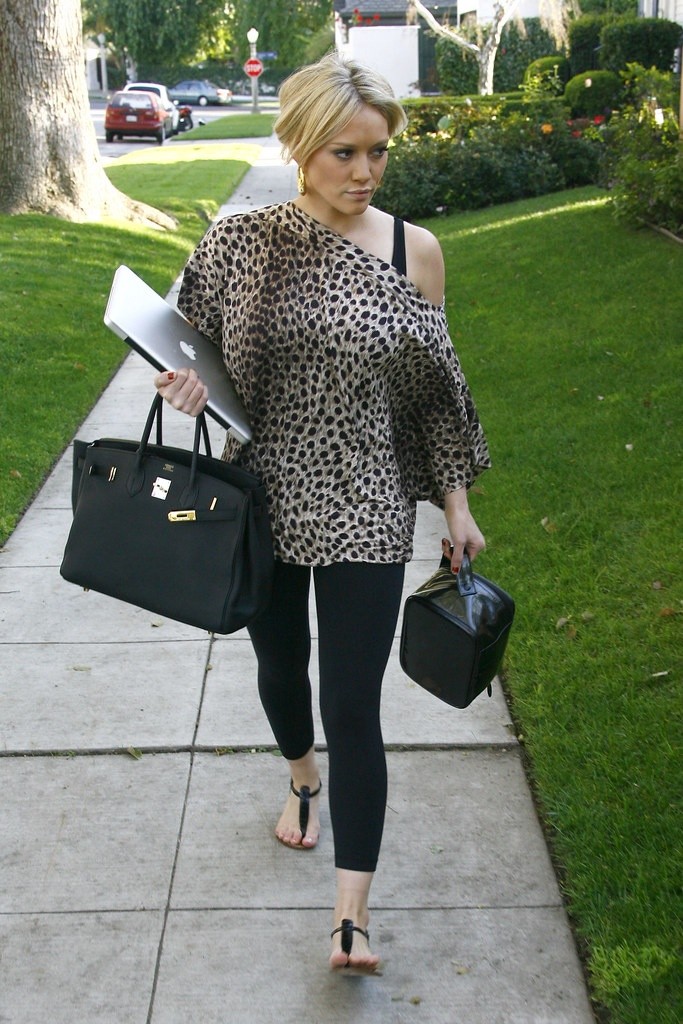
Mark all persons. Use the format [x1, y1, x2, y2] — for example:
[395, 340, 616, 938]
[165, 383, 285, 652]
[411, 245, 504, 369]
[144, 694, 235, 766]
[155, 58, 492, 978]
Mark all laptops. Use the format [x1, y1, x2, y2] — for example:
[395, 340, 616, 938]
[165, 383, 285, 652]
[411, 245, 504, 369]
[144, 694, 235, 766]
[104, 264, 252, 445]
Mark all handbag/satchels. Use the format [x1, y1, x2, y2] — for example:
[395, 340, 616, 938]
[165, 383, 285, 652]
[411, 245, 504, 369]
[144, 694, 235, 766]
[60, 390, 276, 635]
[400, 546, 516, 710]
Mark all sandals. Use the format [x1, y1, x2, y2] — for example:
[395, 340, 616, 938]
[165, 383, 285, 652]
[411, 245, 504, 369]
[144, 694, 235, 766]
[275, 779, 322, 850]
[329, 916, 383, 979]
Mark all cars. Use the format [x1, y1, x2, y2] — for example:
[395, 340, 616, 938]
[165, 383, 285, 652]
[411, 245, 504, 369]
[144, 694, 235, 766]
[167, 79, 234, 106]
[104, 90, 172, 145]
[119, 82, 182, 135]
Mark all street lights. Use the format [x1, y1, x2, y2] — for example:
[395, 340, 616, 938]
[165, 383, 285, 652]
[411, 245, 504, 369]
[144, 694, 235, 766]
[247, 27, 261, 115]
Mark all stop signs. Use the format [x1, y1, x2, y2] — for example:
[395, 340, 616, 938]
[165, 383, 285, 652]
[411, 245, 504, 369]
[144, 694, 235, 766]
[244, 58, 263, 76]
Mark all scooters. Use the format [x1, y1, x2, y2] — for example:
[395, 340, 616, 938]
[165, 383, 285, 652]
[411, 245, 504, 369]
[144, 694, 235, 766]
[173, 98, 194, 133]
